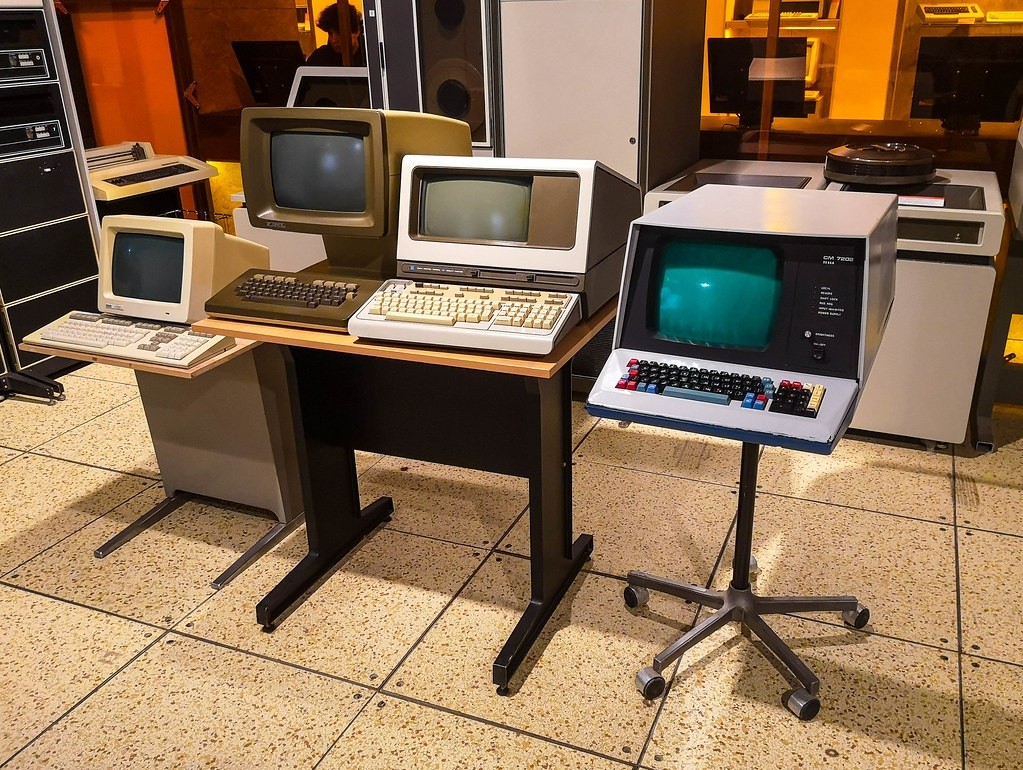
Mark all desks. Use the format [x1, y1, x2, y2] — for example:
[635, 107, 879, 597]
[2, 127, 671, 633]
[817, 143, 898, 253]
[17, 303, 308, 593]
[190, 262, 621, 698]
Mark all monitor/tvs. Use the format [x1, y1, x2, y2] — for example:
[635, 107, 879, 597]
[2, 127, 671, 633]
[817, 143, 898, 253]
[395, 155, 642, 321]
[98, 215, 270, 326]
[648, 242, 783, 349]
[241, 106, 472, 283]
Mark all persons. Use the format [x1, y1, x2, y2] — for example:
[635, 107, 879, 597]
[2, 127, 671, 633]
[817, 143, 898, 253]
[306, 2, 367, 67]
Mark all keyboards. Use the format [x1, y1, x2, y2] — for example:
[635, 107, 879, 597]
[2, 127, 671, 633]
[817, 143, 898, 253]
[615, 358, 826, 420]
[204, 268, 384, 333]
[347, 278, 582, 355]
[22, 311, 238, 370]
